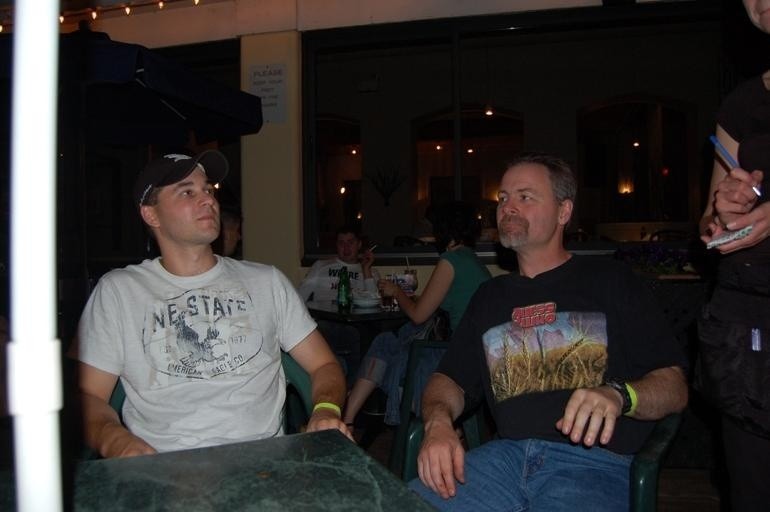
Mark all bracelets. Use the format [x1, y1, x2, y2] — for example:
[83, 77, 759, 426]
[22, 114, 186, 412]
[311, 401, 342, 415]
[621, 382, 640, 418]
[599, 374, 633, 414]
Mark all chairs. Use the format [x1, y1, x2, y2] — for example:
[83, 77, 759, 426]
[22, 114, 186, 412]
[80, 350, 317, 458]
[398, 413, 685, 512]
[387, 340, 451, 473]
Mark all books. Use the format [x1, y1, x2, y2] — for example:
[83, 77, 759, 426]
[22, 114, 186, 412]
[706, 224, 752, 249]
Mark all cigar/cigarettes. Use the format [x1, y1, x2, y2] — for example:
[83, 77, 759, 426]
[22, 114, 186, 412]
[368, 245, 377, 252]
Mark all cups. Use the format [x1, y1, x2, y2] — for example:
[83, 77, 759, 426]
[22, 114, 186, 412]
[379, 289, 393, 309]
[405, 270, 419, 291]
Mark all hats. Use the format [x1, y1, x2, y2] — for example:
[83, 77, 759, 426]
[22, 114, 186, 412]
[134, 149, 229, 211]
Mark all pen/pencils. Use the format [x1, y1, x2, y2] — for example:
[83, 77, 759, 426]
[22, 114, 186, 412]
[709, 136, 762, 197]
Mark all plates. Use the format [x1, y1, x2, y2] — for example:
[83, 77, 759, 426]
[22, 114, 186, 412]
[353, 296, 380, 308]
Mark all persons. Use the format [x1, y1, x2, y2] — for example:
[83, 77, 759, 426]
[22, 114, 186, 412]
[335, 199, 496, 433]
[207, 211, 243, 259]
[694, 2, 769, 511]
[396, 147, 690, 510]
[60, 146, 364, 460]
[296, 222, 383, 324]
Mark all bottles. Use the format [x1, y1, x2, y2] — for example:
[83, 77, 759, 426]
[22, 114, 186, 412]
[337, 266, 352, 310]
